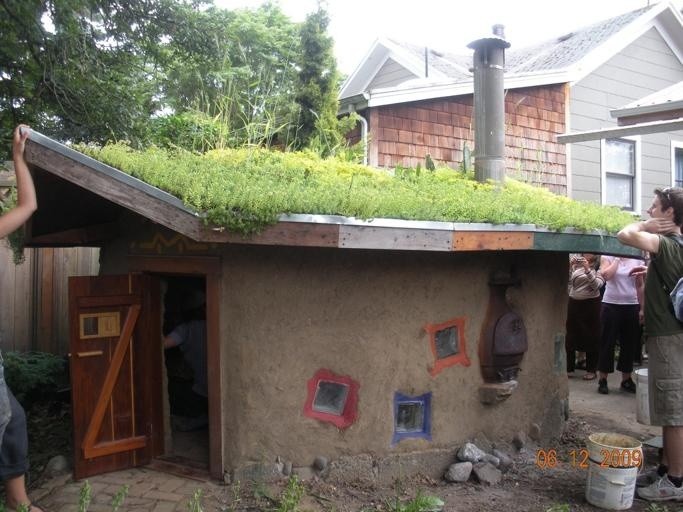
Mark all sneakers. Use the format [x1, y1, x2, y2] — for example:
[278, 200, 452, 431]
[615, 350, 648, 366]
[637, 473, 683, 502]
[621, 378, 636, 394]
[598, 379, 609, 394]
[636, 464, 662, 486]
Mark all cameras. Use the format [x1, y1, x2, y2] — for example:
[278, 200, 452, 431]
[575, 258, 583, 264]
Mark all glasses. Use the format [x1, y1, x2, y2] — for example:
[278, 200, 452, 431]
[662, 186, 673, 206]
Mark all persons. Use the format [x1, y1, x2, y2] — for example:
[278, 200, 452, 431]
[161, 279, 209, 430]
[0, 123, 44, 511]
[562, 184, 683, 501]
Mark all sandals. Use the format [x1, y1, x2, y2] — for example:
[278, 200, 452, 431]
[567, 359, 597, 380]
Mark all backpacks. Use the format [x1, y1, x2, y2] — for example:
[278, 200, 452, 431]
[650, 235, 683, 324]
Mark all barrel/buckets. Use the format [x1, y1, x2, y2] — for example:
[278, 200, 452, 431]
[585, 432, 644, 511]
[632, 368, 651, 424]
